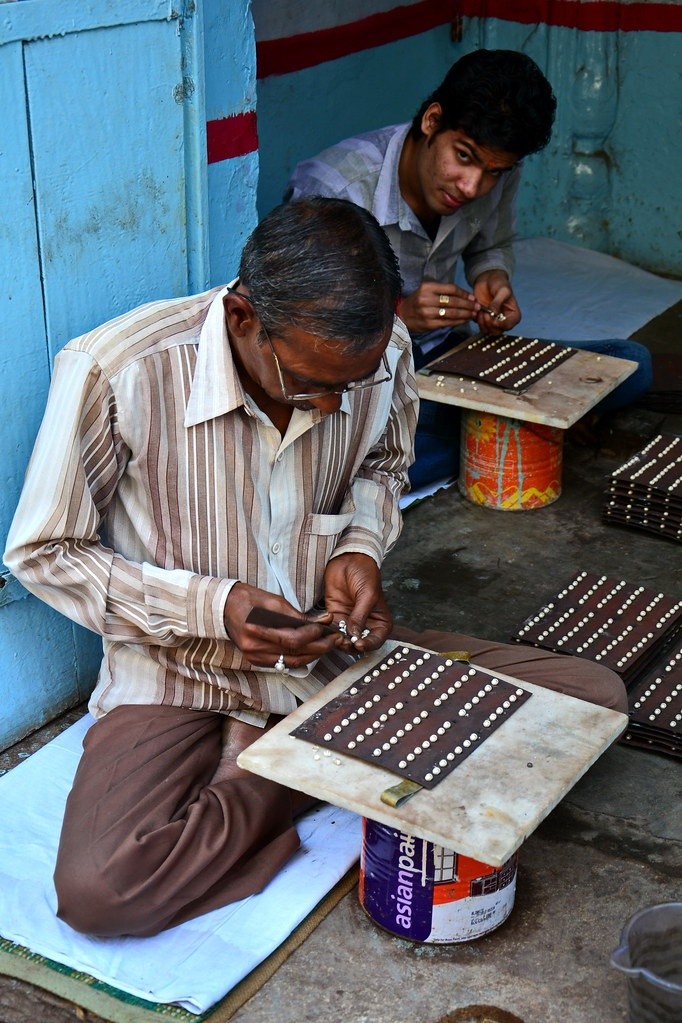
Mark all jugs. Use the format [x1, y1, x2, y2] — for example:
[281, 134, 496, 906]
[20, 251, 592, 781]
[610, 902, 682, 1023]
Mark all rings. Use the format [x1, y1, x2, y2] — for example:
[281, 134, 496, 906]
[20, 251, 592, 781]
[274, 654, 285, 673]
[439, 295, 450, 308]
[439, 307, 447, 319]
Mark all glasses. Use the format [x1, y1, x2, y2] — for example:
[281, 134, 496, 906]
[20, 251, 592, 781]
[226, 286, 392, 400]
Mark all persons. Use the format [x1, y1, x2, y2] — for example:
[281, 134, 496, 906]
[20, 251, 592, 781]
[285, 48, 653, 492]
[0, 197, 632, 947]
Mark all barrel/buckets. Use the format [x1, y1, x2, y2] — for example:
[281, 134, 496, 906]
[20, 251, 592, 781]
[356, 813, 521, 946]
[458, 406, 566, 511]
